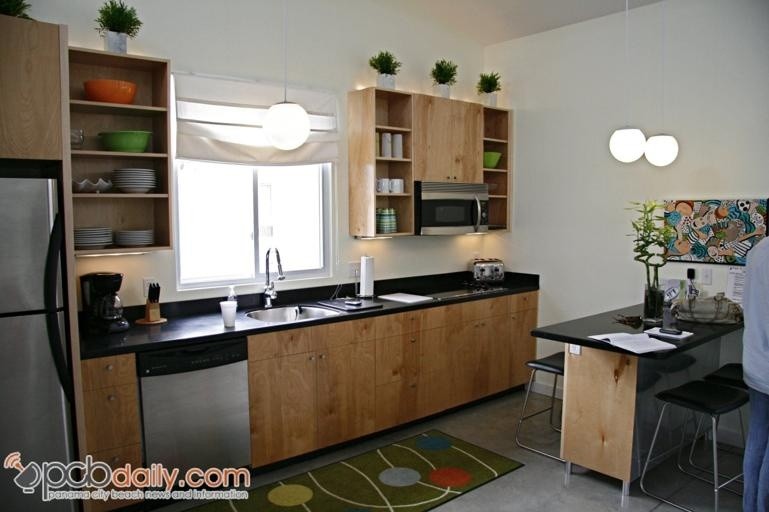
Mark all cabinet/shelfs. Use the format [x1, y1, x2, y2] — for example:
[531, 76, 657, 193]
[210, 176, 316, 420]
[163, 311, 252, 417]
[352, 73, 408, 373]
[451, 294, 508, 409]
[414, 94, 485, 184]
[350, 90, 413, 236]
[0, 15, 64, 162]
[376, 303, 450, 435]
[66, 45, 175, 257]
[80, 353, 143, 511]
[248, 316, 374, 472]
[483, 107, 513, 232]
[510, 289, 539, 388]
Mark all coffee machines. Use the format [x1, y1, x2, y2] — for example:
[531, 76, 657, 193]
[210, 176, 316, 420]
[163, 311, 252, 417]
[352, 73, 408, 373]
[79, 272, 130, 335]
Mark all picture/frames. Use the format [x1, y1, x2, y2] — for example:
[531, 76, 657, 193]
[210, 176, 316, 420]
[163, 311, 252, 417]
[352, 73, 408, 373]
[663, 197, 769, 268]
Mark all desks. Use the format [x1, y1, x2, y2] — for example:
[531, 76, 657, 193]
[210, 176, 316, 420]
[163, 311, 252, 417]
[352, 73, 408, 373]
[529, 299, 745, 498]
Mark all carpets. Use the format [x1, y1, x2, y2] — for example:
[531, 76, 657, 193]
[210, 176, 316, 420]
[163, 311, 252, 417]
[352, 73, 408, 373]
[185, 426, 525, 512]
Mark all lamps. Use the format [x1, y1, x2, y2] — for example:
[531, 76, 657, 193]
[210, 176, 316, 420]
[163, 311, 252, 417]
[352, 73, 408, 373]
[263, 2, 312, 151]
[607, 4, 648, 165]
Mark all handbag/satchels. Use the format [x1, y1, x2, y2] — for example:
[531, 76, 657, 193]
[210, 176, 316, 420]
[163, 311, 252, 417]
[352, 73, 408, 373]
[670, 293, 743, 326]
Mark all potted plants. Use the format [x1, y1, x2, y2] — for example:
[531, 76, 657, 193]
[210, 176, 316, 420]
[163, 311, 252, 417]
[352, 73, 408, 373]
[369, 47, 405, 90]
[622, 200, 679, 320]
[426, 57, 461, 98]
[93, 2, 142, 51]
[475, 71, 504, 108]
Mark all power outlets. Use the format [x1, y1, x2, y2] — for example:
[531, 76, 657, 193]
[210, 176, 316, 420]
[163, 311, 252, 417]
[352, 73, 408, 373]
[700, 267, 713, 285]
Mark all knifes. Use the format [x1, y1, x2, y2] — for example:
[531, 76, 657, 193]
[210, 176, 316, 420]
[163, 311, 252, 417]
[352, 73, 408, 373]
[145, 283, 162, 322]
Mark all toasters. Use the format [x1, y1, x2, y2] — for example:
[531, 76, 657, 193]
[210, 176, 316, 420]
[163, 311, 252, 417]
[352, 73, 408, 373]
[473, 257, 504, 284]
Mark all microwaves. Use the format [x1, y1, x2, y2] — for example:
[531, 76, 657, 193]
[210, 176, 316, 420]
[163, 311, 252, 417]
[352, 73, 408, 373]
[413, 181, 489, 236]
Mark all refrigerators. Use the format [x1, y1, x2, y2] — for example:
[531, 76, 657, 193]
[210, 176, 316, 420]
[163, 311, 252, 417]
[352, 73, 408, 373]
[0, 177, 79, 512]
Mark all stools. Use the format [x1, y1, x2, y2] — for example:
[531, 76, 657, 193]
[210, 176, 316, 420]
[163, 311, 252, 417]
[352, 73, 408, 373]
[516, 351, 576, 464]
[687, 362, 759, 484]
[639, 378, 749, 512]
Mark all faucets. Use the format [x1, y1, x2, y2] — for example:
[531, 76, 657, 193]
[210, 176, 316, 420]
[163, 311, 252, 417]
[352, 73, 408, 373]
[262, 246, 285, 307]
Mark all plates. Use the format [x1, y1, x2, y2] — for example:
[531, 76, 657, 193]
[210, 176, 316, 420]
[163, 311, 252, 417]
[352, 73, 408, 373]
[73, 178, 112, 193]
[114, 228, 156, 247]
[75, 227, 114, 249]
[111, 169, 158, 193]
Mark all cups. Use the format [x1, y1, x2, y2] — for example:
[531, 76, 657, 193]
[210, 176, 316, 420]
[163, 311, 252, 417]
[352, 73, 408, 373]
[389, 179, 405, 193]
[380, 208, 390, 234]
[376, 208, 382, 234]
[377, 178, 389, 194]
[389, 207, 398, 233]
[72, 129, 87, 150]
[219, 300, 238, 328]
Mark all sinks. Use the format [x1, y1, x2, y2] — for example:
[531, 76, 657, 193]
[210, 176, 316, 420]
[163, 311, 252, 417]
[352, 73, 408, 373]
[245, 305, 341, 324]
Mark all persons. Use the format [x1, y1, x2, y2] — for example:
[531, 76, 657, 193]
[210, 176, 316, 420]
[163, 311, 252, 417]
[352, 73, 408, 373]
[738, 235, 769, 512]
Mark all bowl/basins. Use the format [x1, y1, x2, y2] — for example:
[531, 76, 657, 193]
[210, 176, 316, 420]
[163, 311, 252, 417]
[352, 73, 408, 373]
[97, 131, 154, 153]
[485, 152, 502, 168]
[84, 80, 137, 104]
